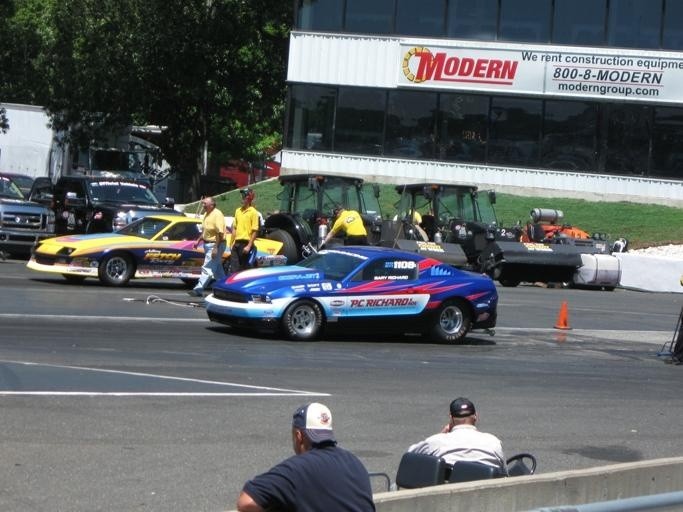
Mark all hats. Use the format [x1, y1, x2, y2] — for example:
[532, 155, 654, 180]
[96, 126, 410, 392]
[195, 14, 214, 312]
[293, 402, 336, 445]
[450, 397, 475, 417]
[242, 188, 253, 200]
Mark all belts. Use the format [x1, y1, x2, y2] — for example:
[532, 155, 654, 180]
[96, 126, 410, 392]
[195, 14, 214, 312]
[204, 241, 214, 244]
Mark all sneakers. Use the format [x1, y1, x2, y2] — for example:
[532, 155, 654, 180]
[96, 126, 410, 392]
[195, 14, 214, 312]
[188, 290, 202, 297]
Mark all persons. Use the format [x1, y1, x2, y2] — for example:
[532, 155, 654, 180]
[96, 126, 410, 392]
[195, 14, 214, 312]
[238, 402, 375, 511]
[229, 193, 259, 273]
[251, 200, 264, 227]
[192, 195, 227, 290]
[407, 397, 508, 477]
[325, 209, 370, 245]
[402, 206, 434, 229]
